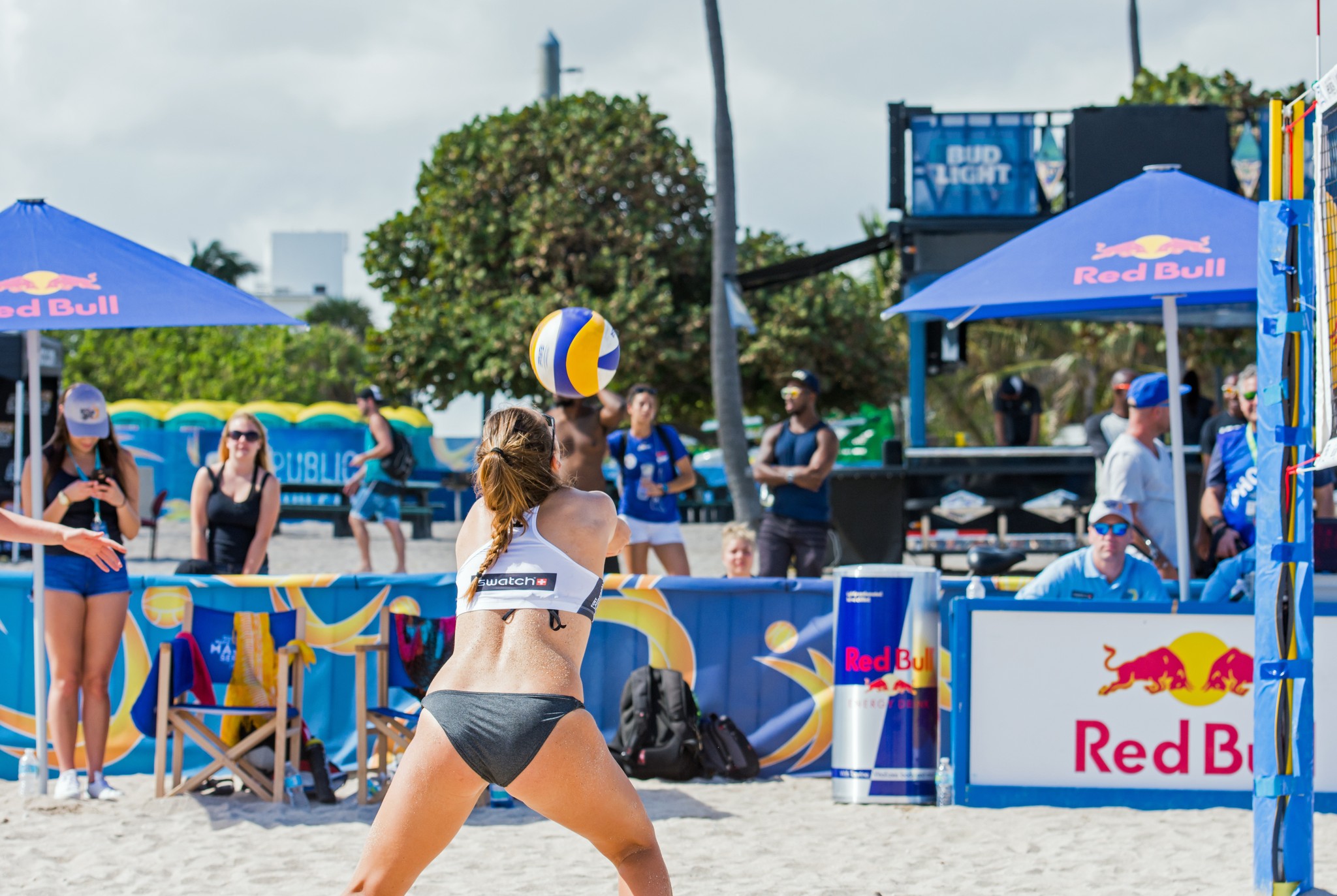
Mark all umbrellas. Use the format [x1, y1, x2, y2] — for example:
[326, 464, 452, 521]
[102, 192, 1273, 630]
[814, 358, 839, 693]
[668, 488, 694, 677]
[879, 162, 1262, 606]
[0, 196, 308, 800]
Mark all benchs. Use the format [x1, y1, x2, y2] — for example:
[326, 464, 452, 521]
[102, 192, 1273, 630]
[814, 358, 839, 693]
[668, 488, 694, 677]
[676, 497, 732, 523]
[272, 499, 448, 537]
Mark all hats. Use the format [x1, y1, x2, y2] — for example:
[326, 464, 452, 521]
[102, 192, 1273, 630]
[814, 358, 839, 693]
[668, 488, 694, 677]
[1088, 499, 1135, 526]
[63, 382, 109, 438]
[356, 385, 385, 402]
[1126, 372, 1192, 407]
[781, 370, 818, 391]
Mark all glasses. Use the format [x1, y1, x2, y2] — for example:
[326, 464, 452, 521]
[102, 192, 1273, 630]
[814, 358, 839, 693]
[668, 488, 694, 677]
[781, 387, 802, 399]
[227, 430, 260, 442]
[1092, 522, 1130, 536]
[1245, 392, 1256, 400]
[1221, 385, 1237, 394]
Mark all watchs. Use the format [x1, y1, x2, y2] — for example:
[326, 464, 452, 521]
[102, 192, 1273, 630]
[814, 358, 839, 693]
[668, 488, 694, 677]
[786, 472, 795, 483]
[59, 490, 71, 505]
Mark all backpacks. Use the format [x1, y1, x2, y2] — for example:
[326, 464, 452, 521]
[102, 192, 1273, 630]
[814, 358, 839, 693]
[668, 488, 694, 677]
[699, 712, 759, 780]
[614, 664, 696, 780]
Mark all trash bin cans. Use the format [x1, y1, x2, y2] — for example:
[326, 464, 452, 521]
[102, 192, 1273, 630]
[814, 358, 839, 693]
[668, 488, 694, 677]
[831, 565, 940, 806]
[829, 469, 904, 565]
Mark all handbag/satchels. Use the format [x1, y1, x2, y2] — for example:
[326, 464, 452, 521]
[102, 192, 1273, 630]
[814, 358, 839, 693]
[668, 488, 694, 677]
[372, 425, 416, 480]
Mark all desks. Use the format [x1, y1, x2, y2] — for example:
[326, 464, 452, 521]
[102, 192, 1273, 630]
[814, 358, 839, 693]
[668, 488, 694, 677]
[281, 479, 440, 539]
[690, 485, 730, 521]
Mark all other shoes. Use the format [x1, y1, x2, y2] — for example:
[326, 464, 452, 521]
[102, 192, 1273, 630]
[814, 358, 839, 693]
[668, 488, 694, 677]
[55, 769, 80, 799]
[85, 770, 124, 799]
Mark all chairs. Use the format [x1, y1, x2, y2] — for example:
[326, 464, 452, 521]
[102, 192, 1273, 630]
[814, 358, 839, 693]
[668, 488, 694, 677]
[355, 606, 462, 802]
[153, 602, 307, 801]
[141, 488, 168, 560]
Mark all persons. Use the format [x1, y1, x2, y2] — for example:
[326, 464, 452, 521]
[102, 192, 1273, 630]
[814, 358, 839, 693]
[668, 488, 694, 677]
[0, 364, 1336, 811]
[343, 409, 678, 896]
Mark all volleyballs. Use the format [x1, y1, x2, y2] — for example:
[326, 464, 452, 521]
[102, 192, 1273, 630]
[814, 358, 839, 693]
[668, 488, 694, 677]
[529, 306, 620, 400]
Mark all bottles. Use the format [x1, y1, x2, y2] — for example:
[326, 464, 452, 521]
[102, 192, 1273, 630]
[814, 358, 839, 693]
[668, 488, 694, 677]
[17, 747, 40, 808]
[935, 756, 953, 806]
[1230, 579, 1248, 604]
[284, 762, 309, 808]
[966, 576, 985, 599]
